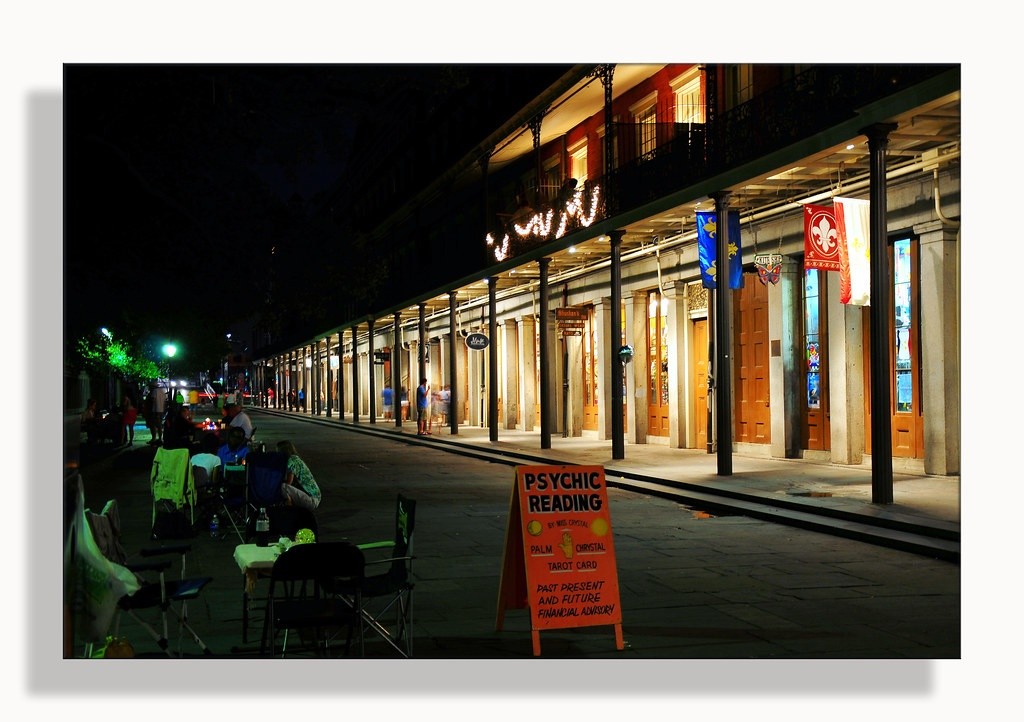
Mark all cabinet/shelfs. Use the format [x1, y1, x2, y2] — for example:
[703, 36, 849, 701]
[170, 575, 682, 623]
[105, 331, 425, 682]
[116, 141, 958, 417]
[805, 278, 913, 412]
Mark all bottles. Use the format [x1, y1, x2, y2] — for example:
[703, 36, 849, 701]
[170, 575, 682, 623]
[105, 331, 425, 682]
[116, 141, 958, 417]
[256, 508, 269, 547]
[210, 515, 219, 537]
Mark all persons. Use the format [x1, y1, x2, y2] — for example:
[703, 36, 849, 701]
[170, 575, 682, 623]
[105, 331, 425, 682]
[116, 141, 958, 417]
[176, 391, 184, 407]
[189, 433, 222, 483]
[417, 378, 451, 435]
[217, 427, 251, 466]
[299, 388, 305, 408]
[177, 402, 253, 448]
[276, 439, 322, 509]
[288, 388, 296, 408]
[381, 384, 410, 423]
[143, 383, 162, 444]
[267, 387, 274, 405]
[187, 387, 199, 417]
[82, 385, 137, 447]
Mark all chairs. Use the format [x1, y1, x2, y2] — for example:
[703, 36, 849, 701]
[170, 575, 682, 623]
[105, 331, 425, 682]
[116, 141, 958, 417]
[246, 451, 286, 521]
[320, 493, 416, 659]
[265, 543, 366, 659]
[85, 499, 216, 659]
[149, 446, 210, 535]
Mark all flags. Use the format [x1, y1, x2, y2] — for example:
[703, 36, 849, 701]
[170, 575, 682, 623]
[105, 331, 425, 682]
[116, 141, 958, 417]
[695, 211, 745, 290]
[803, 197, 871, 307]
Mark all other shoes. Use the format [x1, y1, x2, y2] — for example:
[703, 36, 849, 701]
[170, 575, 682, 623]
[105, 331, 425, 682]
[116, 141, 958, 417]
[146, 439, 153, 444]
[418, 431, 428, 435]
[152, 440, 162, 445]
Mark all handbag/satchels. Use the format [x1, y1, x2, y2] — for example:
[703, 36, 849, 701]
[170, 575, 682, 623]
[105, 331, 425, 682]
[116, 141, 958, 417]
[64, 473, 142, 644]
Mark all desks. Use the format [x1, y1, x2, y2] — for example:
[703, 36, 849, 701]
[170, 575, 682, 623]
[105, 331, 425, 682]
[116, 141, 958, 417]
[223, 464, 247, 534]
[233, 544, 352, 656]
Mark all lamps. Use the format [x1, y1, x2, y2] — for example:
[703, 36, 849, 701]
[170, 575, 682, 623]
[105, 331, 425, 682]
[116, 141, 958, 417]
[618, 344, 635, 379]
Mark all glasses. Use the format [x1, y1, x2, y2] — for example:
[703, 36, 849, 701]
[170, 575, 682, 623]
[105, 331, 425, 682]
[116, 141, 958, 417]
[231, 434, 243, 439]
